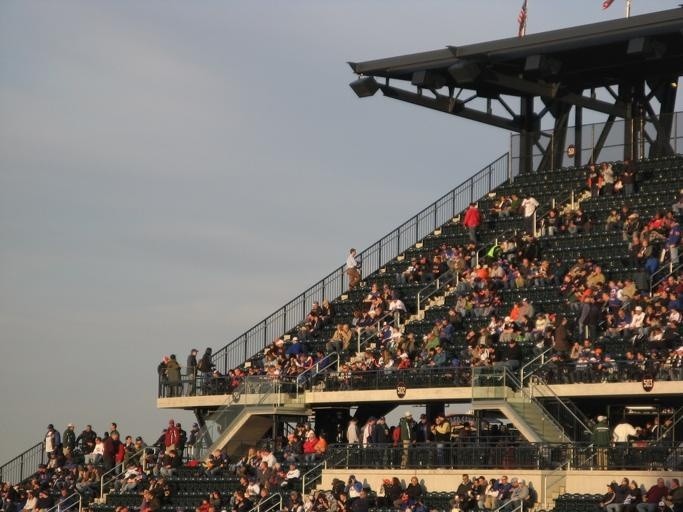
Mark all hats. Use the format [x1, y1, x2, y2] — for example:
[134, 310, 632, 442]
[175, 423, 181, 427]
[67, 423, 74, 428]
[136, 436, 142, 440]
[192, 423, 198, 427]
[47, 424, 53, 429]
[191, 348, 199, 352]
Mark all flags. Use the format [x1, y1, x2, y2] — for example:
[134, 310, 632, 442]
[601, 0, 616, 12]
[516, 0, 528, 39]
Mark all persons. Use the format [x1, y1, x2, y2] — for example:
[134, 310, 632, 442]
[538, 208, 589, 235]
[607, 202, 682, 286]
[592, 414, 677, 470]
[491, 193, 539, 229]
[588, 158, 637, 201]
[158, 232, 682, 397]
[0, 420, 202, 512]
[464, 203, 481, 240]
[203, 414, 532, 511]
[600, 478, 683, 511]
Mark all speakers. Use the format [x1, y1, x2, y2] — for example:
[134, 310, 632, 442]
[412, 71, 432, 89]
[349, 77, 379, 98]
[625, 37, 666, 65]
[524, 55, 561, 80]
[448, 61, 480, 84]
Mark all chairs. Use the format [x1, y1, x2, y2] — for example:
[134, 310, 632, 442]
[6, 408, 681, 508]
[205, 149, 683, 395]
